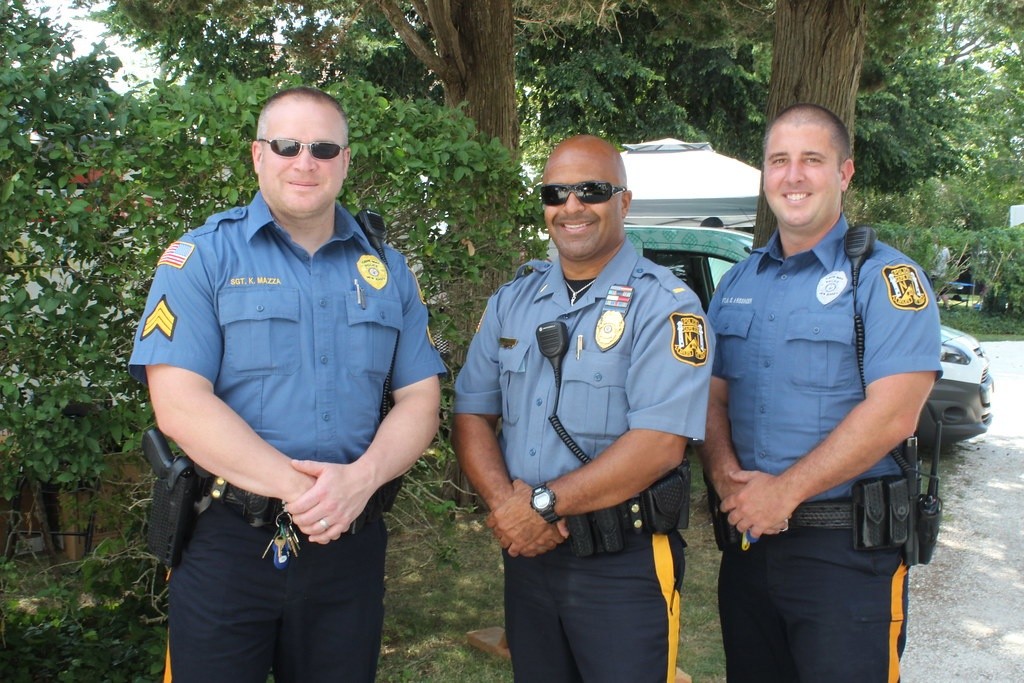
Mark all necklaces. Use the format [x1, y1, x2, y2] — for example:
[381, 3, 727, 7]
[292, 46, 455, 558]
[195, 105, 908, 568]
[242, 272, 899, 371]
[562, 278, 597, 307]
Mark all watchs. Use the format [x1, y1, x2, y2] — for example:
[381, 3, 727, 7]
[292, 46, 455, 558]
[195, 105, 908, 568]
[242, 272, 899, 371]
[531, 482, 560, 523]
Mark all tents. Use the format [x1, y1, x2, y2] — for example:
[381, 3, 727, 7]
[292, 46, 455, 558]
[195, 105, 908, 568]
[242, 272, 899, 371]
[618, 137, 764, 231]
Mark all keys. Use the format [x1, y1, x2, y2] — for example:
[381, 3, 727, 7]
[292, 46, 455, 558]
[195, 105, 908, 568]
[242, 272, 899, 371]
[261, 522, 301, 561]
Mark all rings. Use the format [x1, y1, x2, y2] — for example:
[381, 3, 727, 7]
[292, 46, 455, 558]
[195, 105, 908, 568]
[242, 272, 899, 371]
[319, 518, 331, 530]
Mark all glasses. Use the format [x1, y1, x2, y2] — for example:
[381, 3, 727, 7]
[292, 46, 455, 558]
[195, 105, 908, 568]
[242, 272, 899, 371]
[257, 137, 345, 161]
[540, 180, 628, 206]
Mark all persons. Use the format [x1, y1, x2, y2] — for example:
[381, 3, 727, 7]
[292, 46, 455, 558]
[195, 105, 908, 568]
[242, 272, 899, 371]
[452, 134, 716, 683]
[701, 105, 944, 682]
[926, 234, 951, 312]
[130, 88, 450, 683]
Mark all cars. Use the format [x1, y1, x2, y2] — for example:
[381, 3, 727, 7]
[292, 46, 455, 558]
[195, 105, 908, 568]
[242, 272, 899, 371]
[622, 226, 995, 455]
